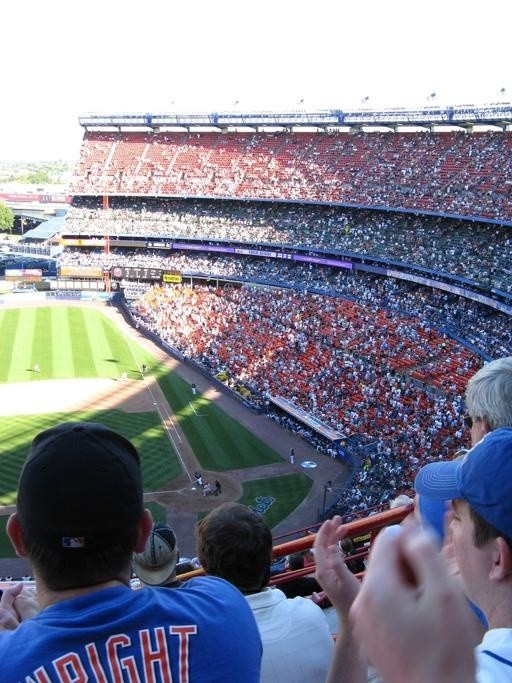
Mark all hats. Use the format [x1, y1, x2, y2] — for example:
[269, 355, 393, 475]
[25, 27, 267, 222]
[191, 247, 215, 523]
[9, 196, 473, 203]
[132, 522, 177, 585]
[415, 427, 512, 536]
[390, 494, 413, 508]
[17, 422, 143, 550]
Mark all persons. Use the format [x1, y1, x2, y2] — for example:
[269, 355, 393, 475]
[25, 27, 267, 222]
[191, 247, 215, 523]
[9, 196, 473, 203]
[0, 127, 512, 683]
